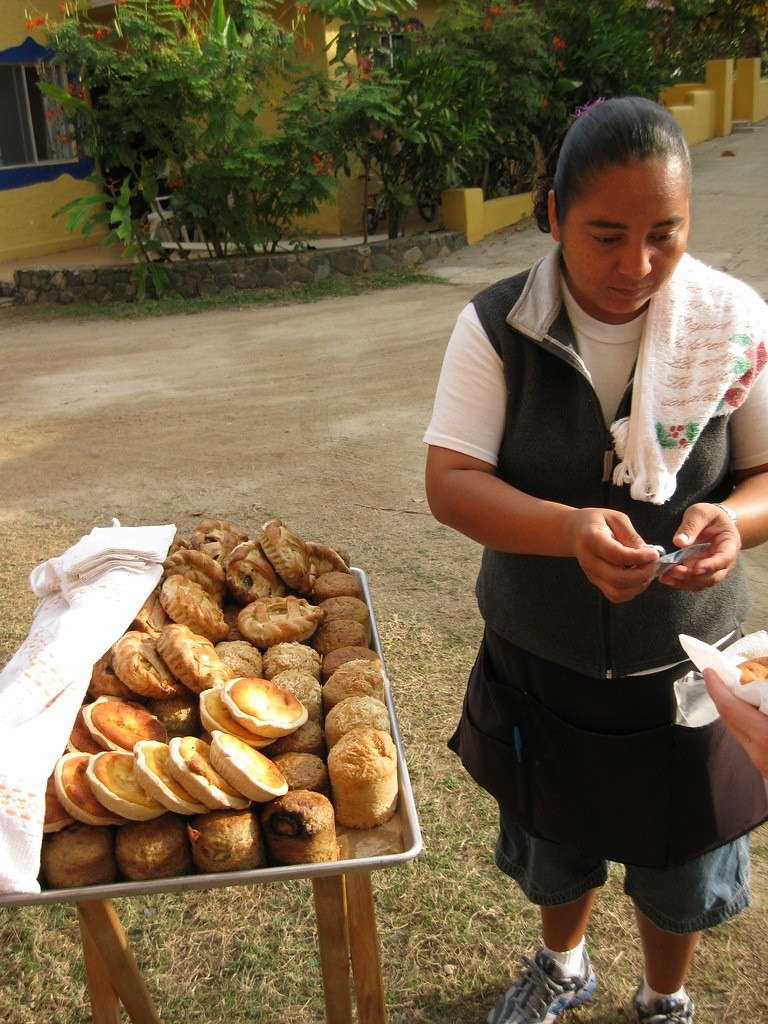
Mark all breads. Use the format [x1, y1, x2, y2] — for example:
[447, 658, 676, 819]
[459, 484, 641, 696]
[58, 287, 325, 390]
[734, 656, 768, 684]
[39, 518, 398, 887]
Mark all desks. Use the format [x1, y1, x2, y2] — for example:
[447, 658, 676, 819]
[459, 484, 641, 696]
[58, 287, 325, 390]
[0, 567, 423, 1024]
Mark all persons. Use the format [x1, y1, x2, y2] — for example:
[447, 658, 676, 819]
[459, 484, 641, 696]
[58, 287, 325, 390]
[422, 97, 768, 1024]
[702, 668, 768, 782]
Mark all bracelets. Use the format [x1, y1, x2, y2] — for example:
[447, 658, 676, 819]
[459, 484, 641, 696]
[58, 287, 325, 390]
[713, 503, 736, 526]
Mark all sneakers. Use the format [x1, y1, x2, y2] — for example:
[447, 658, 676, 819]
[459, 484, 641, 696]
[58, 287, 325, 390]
[632, 979, 695, 1024]
[487, 947, 598, 1024]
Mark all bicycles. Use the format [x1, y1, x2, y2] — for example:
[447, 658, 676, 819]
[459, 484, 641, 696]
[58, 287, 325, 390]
[356, 173, 435, 234]
